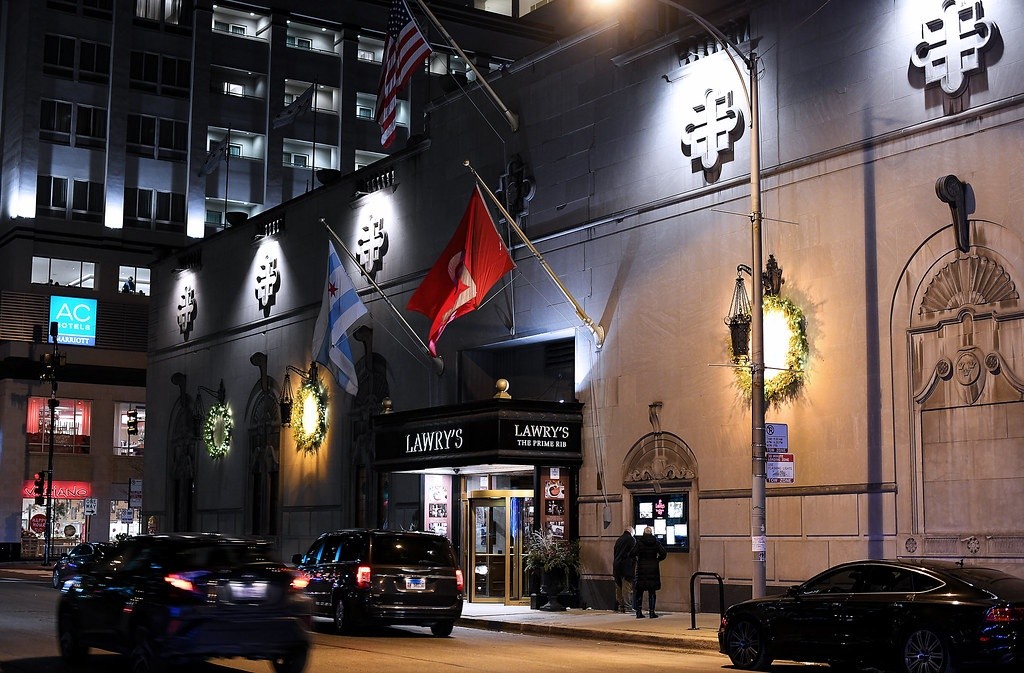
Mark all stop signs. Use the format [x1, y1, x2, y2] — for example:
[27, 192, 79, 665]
[30, 514, 47, 533]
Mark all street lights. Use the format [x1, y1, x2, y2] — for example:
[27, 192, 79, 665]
[44, 320, 60, 566]
[581, 0, 766, 604]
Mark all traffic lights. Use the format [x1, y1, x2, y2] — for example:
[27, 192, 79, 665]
[34, 471, 45, 506]
[127, 410, 138, 435]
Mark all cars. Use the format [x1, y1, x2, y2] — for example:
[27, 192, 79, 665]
[53, 541, 113, 590]
[717, 558, 1024, 673]
[57, 535, 316, 673]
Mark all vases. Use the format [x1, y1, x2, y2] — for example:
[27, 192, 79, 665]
[536, 563, 565, 612]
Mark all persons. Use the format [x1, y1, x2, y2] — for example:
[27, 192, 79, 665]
[613, 526, 636, 614]
[629, 526, 667, 619]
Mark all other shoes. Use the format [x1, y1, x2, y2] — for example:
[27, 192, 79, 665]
[650, 614, 657, 618]
[619, 610, 624, 613]
[636, 612, 645, 618]
[625, 609, 636, 614]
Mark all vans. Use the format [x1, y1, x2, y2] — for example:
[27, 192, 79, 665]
[291, 527, 464, 639]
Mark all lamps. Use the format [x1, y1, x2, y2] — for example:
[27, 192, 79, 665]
[171, 268, 186, 273]
[278, 357, 319, 430]
[724, 253, 786, 359]
[191, 376, 225, 425]
[351, 191, 372, 198]
[251, 235, 268, 241]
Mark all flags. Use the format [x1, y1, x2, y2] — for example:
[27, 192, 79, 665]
[198, 135, 227, 177]
[375, 0, 432, 148]
[310, 241, 368, 396]
[273, 84, 314, 130]
[405, 182, 518, 357]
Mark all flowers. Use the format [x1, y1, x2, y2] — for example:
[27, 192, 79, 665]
[520, 527, 584, 580]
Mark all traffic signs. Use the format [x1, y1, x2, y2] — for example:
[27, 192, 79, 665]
[121, 509, 134, 524]
[130, 479, 143, 507]
[84, 498, 97, 516]
